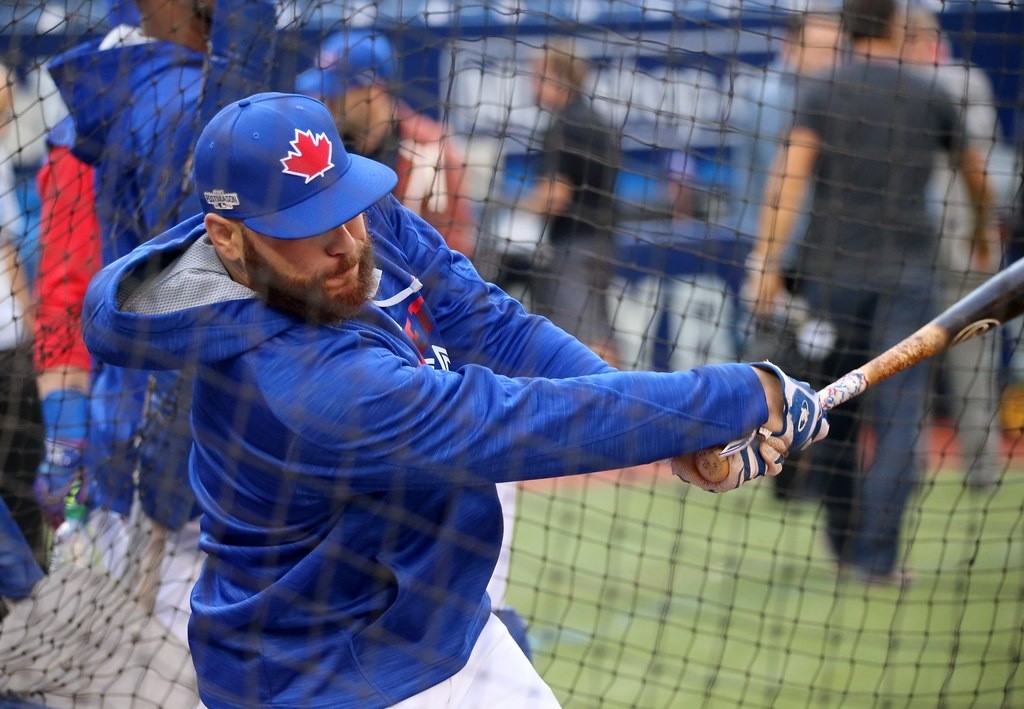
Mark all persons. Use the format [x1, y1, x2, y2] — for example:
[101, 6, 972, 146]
[0, 1, 521, 708]
[82, 92, 830, 709]
[736, 1, 1024, 588]
[656, 149, 708, 227]
[513, 42, 624, 367]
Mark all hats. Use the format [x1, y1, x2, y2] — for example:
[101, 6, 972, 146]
[192, 93, 398, 241]
[294, 27, 399, 97]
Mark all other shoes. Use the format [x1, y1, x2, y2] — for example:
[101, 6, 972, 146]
[850, 567, 916, 590]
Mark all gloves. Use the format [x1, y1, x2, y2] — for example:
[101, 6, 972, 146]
[32, 438, 90, 543]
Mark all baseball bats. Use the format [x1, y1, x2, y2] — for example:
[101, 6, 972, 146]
[694, 254, 1024, 484]
